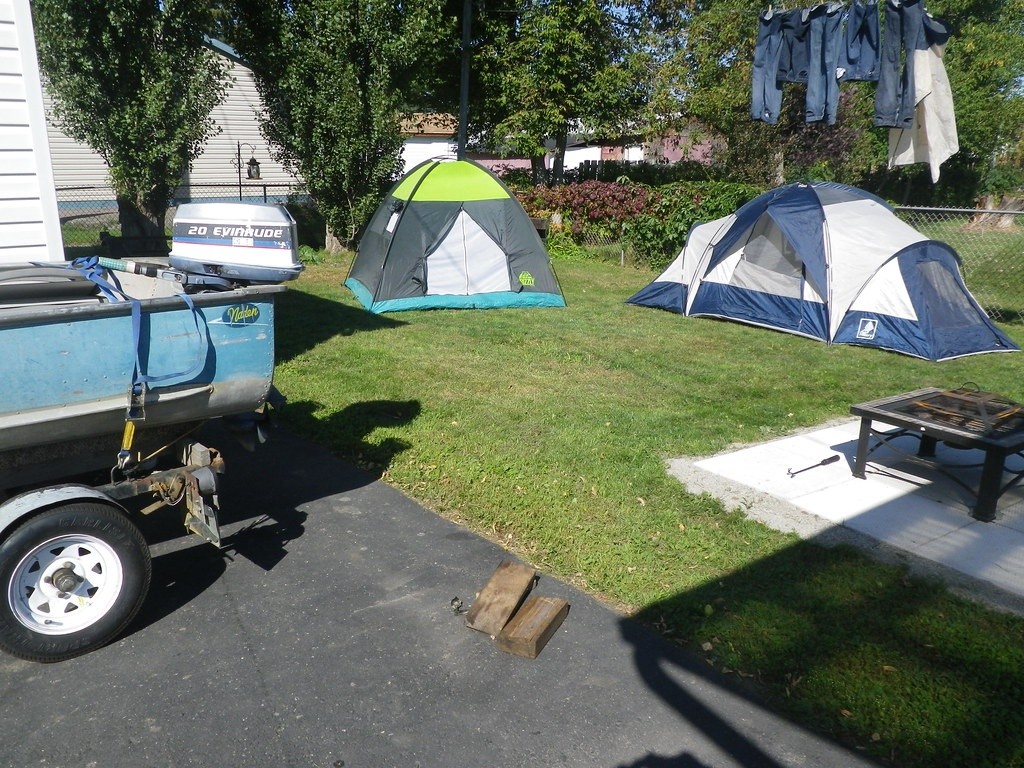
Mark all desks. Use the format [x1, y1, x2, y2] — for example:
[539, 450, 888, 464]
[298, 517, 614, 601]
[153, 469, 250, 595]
[848, 387, 1024, 523]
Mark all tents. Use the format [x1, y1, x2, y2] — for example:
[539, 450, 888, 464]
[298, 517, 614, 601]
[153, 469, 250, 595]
[626, 185, 1023, 364]
[343, 155, 569, 314]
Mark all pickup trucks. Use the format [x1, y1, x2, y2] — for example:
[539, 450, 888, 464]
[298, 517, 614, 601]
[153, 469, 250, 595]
[0, 196, 304, 663]
